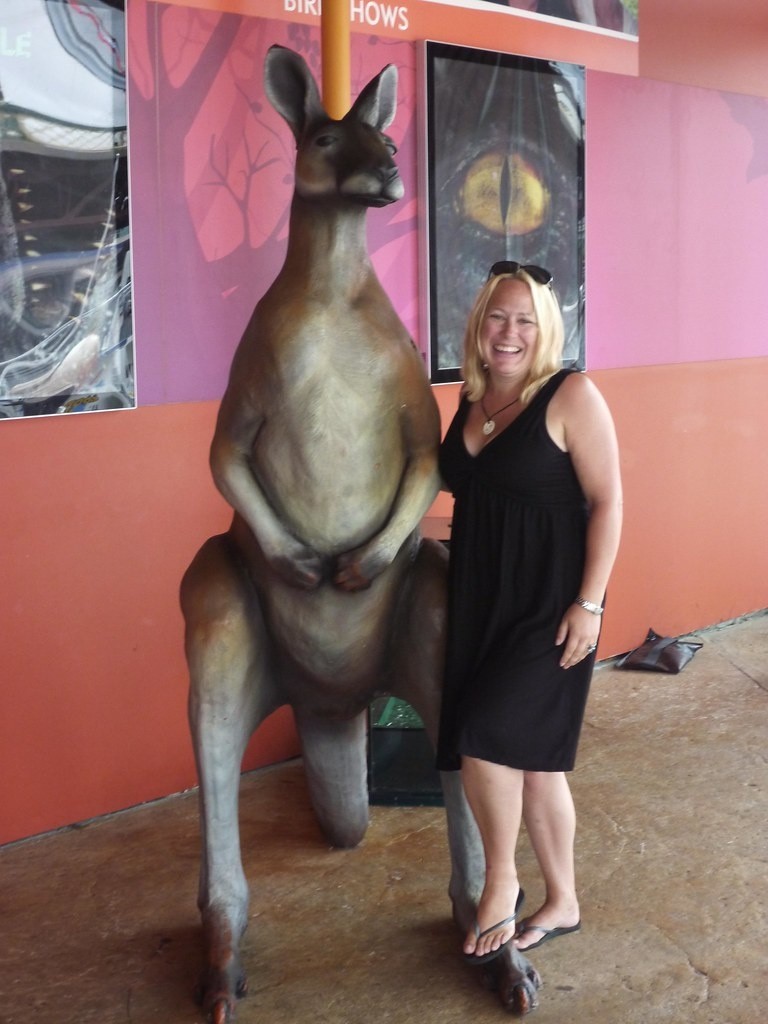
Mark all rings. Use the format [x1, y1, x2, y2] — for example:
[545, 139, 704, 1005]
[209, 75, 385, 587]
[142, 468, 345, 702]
[587, 645, 596, 652]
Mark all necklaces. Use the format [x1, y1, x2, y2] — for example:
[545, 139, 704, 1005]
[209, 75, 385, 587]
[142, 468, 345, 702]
[481, 396, 520, 435]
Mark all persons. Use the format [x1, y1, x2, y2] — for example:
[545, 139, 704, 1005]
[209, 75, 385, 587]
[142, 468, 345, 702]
[438, 259, 624, 966]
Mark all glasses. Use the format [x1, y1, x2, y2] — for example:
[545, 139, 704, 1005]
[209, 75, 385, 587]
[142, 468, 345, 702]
[487, 261, 553, 291]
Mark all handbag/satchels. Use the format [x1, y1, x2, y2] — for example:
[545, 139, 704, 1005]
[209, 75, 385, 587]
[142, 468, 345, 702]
[615, 627, 703, 674]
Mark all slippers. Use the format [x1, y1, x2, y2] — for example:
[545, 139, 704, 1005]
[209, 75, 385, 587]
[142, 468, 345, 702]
[517, 918, 581, 952]
[463, 887, 525, 963]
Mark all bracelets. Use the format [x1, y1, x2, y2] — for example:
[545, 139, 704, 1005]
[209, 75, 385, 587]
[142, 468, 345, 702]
[577, 596, 604, 615]
